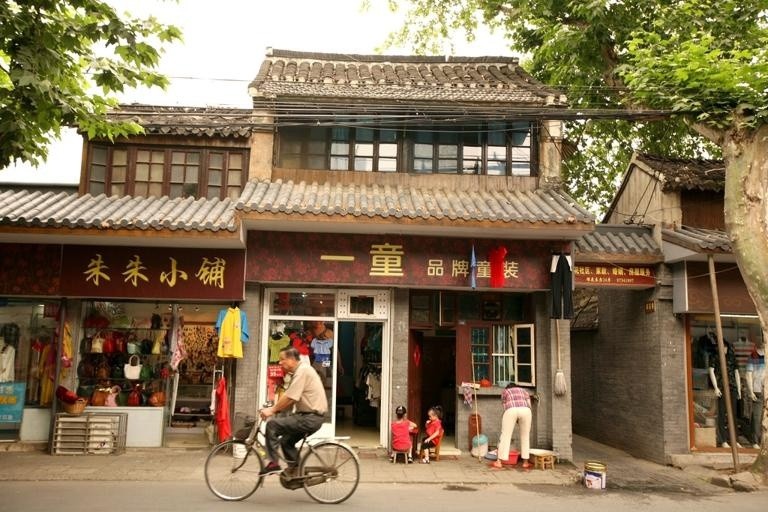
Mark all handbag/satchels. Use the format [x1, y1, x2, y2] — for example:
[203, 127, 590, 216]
[205, 420, 219, 445]
[80, 331, 162, 355]
[78, 384, 165, 406]
[109, 314, 131, 329]
[84, 313, 109, 329]
[77, 355, 171, 380]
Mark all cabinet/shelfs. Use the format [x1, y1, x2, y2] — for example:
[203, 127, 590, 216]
[173, 367, 215, 419]
[80, 321, 168, 382]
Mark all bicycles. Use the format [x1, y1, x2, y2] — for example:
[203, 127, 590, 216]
[204, 399, 360, 505]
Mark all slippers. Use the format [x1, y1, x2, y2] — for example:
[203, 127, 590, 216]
[488, 462, 502, 468]
[522, 463, 533, 468]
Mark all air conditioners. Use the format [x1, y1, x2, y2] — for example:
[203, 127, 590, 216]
[54, 415, 120, 454]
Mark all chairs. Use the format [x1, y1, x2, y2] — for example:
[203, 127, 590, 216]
[419, 429, 445, 462]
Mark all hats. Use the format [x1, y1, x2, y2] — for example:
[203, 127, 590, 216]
[755, 344, 765, 356]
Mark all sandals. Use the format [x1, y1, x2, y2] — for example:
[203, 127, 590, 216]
[419, 458, 430, 464]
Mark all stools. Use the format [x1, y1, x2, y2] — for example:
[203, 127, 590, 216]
[528, 448, 555, 471]
[392, 450, 409, 465]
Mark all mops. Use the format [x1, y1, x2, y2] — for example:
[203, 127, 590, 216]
[553, 319, 567, 397]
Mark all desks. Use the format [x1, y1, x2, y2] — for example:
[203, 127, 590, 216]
[409, 427, 420, 461]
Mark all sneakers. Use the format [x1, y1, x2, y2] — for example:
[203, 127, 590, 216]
[259, 462, 283, 477]
[407, 457, 415, 463]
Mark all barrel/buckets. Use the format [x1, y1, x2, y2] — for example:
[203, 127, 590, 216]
[582, 459, 607, 490]
[232, 435, 248, 459]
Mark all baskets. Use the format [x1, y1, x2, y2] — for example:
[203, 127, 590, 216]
[232, 412, 257, 440]
[57, 396, 88, 414]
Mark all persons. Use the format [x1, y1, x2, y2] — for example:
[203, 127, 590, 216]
[389, 405, 416, 461]
[307, 312, 345, 376]
[486, 382, 536, 468]
[707, 346, 744, 448]
[745, 342, 766, 447]
[419, 405, 443, 463]
[259, 345, 328, 476]
[0, 333, 16, 383]
